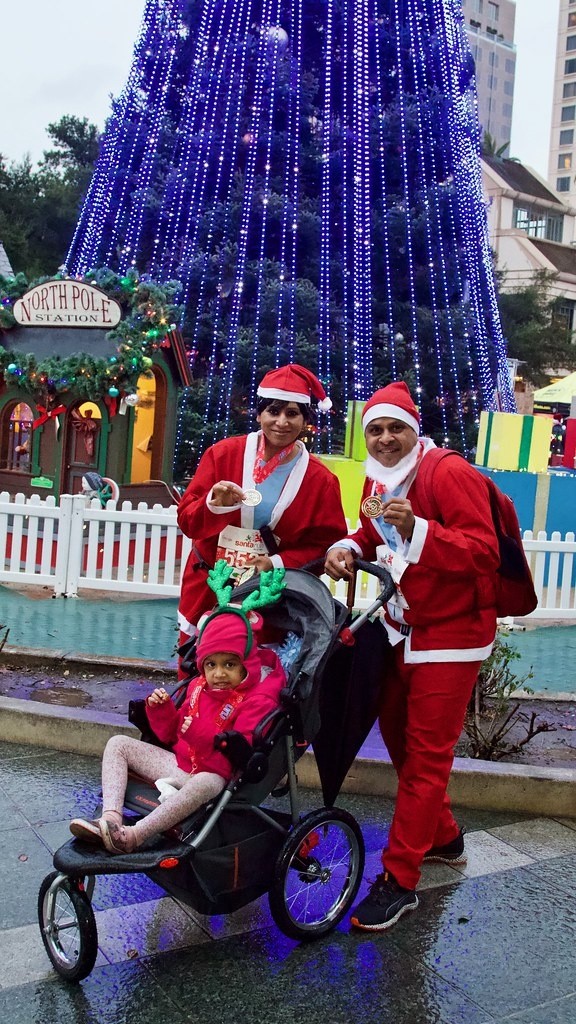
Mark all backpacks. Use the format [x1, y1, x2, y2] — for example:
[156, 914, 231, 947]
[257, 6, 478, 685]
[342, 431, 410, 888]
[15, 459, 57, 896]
[416, 448, 538, 617]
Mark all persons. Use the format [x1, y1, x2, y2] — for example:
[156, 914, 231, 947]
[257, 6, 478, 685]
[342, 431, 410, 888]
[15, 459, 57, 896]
[175, 364, 349, 678]
[325, 382, 503, 931]
[69, 607, 287, 855]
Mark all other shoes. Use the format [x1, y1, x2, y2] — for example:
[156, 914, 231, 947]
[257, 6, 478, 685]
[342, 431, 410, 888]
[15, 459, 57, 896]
[70, 818, 101, 843]
[99, 819, 134, 853]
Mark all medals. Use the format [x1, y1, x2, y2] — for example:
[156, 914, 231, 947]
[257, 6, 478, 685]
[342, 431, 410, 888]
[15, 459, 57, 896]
[361, 496, 384, 519]
[241, 489, 262, 506]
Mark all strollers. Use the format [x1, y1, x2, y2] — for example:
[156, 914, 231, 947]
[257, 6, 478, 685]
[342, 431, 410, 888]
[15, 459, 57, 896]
[37, 558, 397, 987]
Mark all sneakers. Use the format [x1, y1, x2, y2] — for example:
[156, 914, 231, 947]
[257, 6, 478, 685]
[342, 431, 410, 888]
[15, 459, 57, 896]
[351, 873, 420, 931]
[381, 824, 468, 865]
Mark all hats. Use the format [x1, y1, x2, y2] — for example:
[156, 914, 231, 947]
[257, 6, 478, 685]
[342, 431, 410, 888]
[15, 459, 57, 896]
[196, 604, 264, 691]
[257, 364, 332, 412]
[362, 381, 420, 438]
